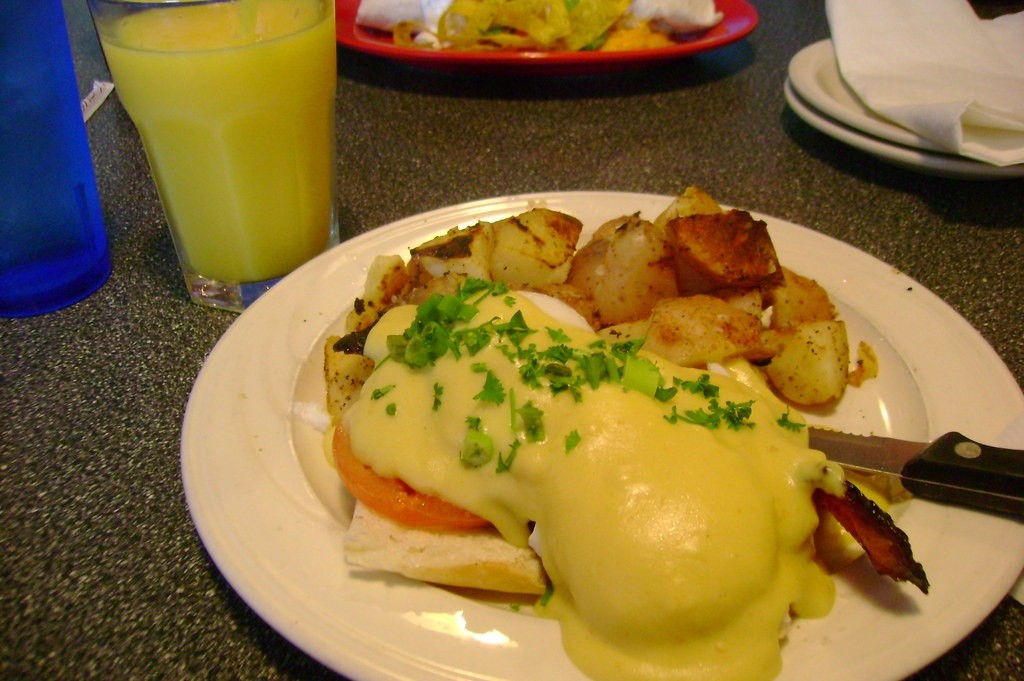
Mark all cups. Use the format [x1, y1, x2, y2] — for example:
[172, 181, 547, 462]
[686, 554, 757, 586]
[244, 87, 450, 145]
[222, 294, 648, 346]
[88, 0, 340, 313]
[0, 0, 112, 319]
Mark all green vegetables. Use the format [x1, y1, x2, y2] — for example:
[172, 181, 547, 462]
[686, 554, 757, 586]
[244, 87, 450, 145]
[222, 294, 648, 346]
[371, 277, 809, 472]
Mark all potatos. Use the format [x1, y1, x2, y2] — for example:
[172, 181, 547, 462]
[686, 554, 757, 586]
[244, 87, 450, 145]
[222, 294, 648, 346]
[326, 185, 851, 406]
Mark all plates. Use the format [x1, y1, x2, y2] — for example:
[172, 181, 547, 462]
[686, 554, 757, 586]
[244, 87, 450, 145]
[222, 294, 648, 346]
[788, 38, 1024, 154]
[335, 0, 758, 64]
[785, 80, 1024, 203]
[181, 191, 1024, 680]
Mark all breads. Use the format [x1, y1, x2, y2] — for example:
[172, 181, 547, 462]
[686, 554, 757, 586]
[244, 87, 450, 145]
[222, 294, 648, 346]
[344, 496, 548, 593]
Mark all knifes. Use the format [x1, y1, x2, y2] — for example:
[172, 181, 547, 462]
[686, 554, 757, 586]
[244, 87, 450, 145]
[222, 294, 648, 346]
[808, 426, 1024, 517]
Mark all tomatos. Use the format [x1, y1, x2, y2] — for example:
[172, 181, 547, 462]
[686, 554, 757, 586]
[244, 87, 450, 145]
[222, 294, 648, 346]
[333, 420, 492, 529]
